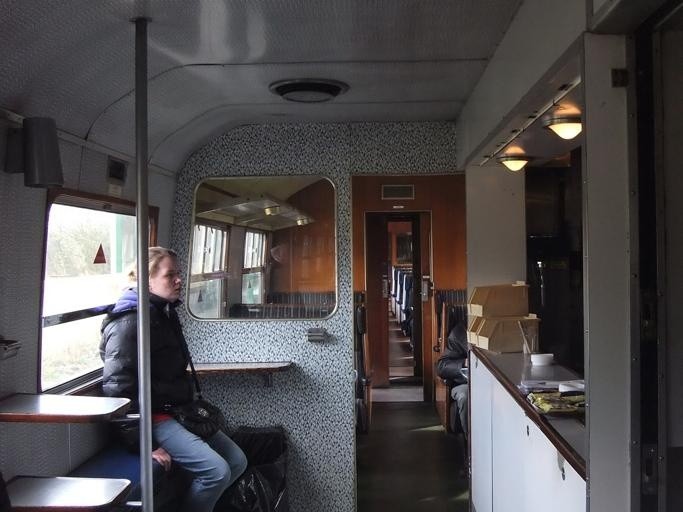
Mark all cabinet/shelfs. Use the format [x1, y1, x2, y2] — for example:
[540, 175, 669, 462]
[0, 391, 131, 512]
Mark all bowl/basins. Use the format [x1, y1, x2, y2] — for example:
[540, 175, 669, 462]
[558, 381, 583, 392]
[530, 353, 554, 366]
[529, 365, 553, 379]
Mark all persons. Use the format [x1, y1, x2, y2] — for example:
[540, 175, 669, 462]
[437, 318, 468, 440]
[98, 244, 249, 511]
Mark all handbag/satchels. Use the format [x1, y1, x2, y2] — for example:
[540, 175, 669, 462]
[173, 397, 225, 440]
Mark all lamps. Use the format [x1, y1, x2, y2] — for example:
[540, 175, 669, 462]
[498, 155, 532, 172]
[541, 117, 582, 140]
[268, 78, 350, 104]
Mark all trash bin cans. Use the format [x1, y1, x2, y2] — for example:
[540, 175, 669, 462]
[214, 425, 290, 512]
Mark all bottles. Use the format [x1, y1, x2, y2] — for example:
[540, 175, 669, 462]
[534, 260, 550, 307]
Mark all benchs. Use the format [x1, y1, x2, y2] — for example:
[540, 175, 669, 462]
[64, 423, 289, 510]
[434, 301, 470, 436]
[225, 304, 370, 433]
[387, 263, 414, 347]
[430, 289, 466, 403]
[265, 291, 371, 389]
